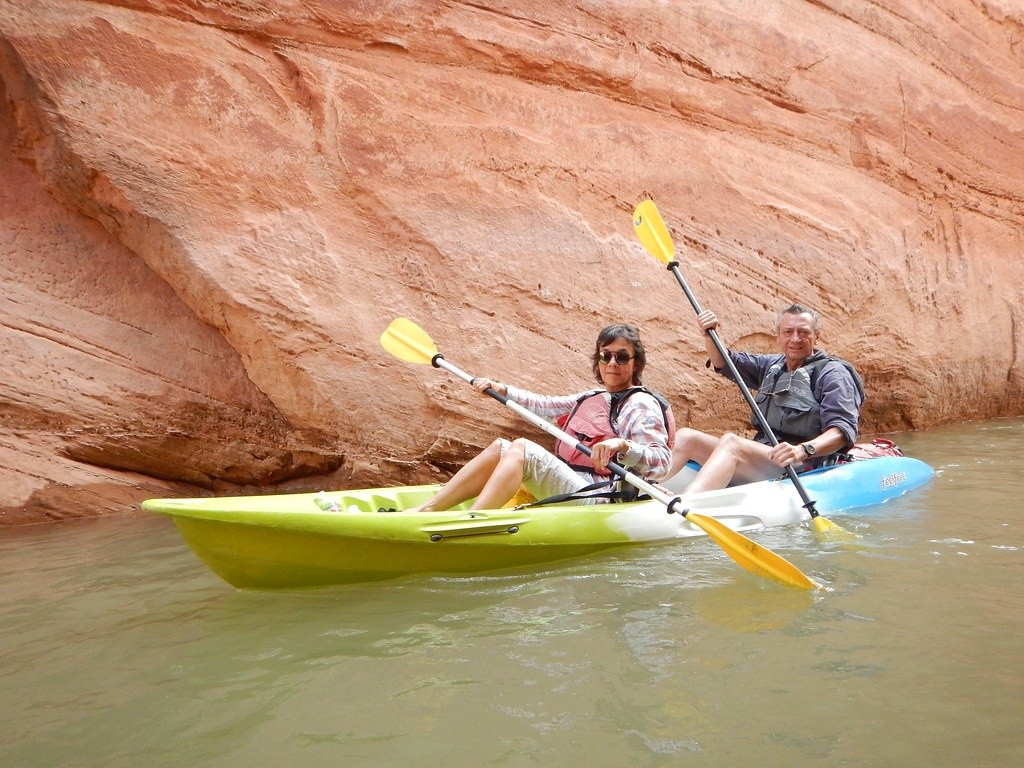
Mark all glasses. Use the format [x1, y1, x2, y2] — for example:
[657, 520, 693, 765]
[598, 351, 636, 365]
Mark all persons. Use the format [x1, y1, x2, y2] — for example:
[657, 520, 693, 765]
[404, 323, 676, 512]
[636, 304, 865, 502]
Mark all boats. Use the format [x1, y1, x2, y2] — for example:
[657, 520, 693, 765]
[137, 452, 935, 590]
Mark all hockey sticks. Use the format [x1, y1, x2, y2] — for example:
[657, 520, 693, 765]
[629, 196, 853, 539]
[377, 316, 821, 591]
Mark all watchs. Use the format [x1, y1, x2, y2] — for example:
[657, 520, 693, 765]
[800, 442, 815, 461]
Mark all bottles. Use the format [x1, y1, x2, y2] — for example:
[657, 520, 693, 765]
[317, 491, 343, 512]
